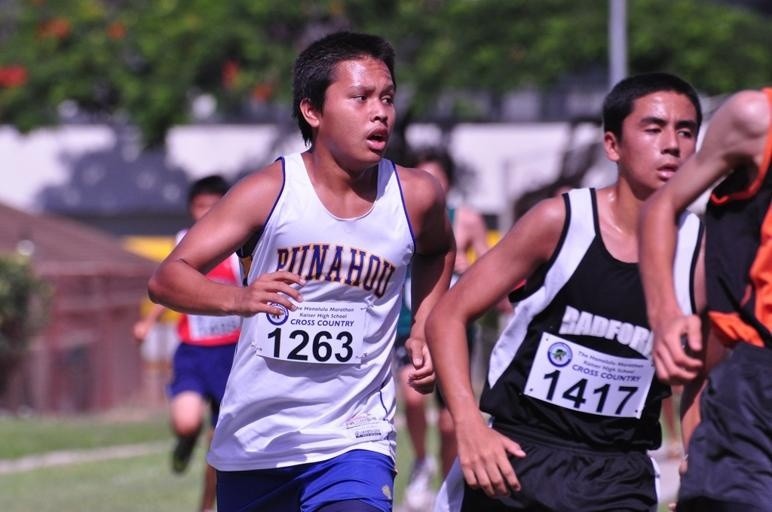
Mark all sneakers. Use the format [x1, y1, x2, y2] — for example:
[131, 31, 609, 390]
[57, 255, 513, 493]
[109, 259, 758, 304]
[405, 460, 432, 509]
[173, 435, 192, 472]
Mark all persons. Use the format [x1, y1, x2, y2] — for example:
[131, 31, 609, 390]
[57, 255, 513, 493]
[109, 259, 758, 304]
[132, 175, 246, 512]
[393, 145, 490, 511]
[638, 88, 771, 510]
[424, 71, 705, 511]
[144, 30, 457, 511]
